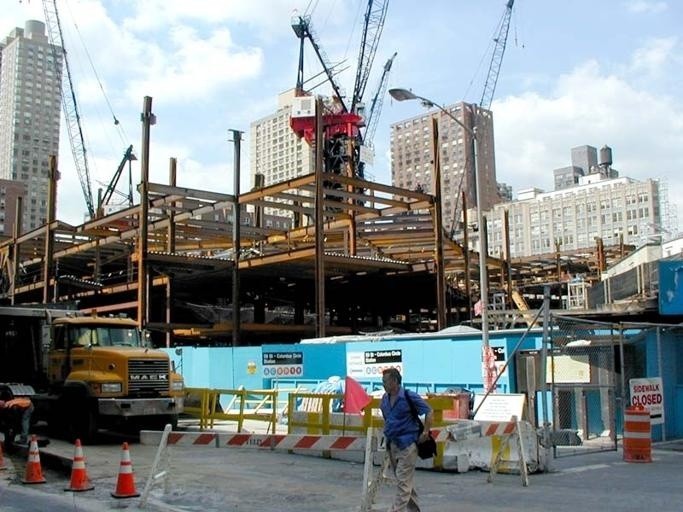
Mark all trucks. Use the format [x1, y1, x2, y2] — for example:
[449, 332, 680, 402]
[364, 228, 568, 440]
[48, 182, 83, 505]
[0, 304, 187, 447]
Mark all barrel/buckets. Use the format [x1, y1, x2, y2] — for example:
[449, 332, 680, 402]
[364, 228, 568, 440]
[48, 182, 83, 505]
[622, 408, 652, 463]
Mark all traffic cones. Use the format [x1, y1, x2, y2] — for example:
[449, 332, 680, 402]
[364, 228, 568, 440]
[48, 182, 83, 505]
[108, 439, 144, 500]
[0, 442, 8, 470]
[60, 435, 95, 493]
[17, 433, 48, 484]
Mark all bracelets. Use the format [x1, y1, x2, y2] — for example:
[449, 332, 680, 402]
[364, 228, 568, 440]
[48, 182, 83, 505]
[422, 432, 428, 436]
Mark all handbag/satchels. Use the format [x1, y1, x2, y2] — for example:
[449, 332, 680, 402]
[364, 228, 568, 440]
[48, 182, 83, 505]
[417, 424, 437, 460]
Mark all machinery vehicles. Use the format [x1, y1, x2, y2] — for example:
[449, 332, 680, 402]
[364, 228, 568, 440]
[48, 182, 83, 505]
[284, 0, 397, 219]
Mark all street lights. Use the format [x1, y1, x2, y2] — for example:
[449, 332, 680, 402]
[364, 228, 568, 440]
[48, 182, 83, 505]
[383, 83, 494, 395]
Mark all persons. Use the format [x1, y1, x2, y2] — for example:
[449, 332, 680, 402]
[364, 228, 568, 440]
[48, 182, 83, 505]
[330, 96, 343, 114]
[0, 397, 35, 445]
[380, 369, 434, 511]
